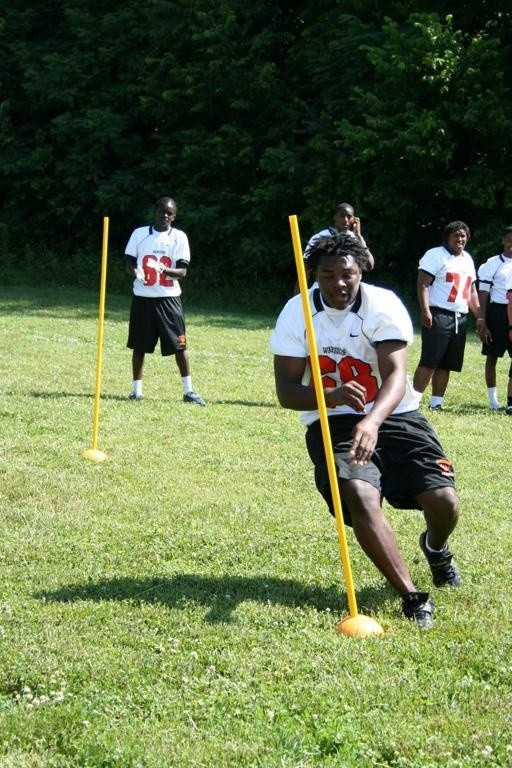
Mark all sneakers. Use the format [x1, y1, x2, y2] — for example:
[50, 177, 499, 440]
[419, 530, 461, 590]
[428, 404, 442, 412]
[127, 392, 144, 401]
[398, 590, 436, 629]
[183, 391, 205, 406]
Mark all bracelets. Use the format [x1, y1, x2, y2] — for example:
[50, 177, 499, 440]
[477, 318, 486, 321]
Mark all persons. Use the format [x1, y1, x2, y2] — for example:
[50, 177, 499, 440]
[504, 262, 512, 414]
[270, 225, 463, 636]
[300, 202, 375, 289]
[123, 195, 208, 407]
[409, 219, 492, 414]
[477, 226, 512, 411]
